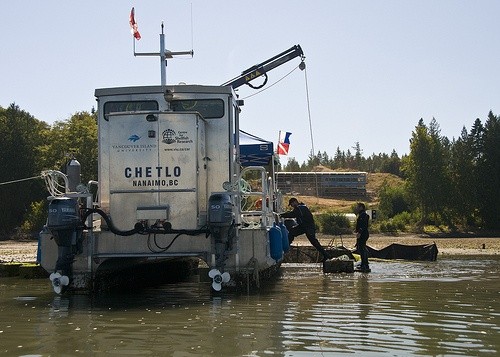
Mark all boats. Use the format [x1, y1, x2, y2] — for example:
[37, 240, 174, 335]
[36, 0, 306, 294]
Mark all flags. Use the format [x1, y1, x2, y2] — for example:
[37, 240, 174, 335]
[278, 132, 292, 156]
[129, 8, 141, 40]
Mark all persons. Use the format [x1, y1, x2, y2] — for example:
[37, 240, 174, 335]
[278, 198, 332, 262]
[353, 203, 371, 273]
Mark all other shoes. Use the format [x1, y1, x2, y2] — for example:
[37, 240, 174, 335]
[320, 254, 331, 262]
[357, 266, 361, 269]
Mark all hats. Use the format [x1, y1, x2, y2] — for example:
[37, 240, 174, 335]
[288, 198, 297, 206]
[357, 202, 365, 209]
